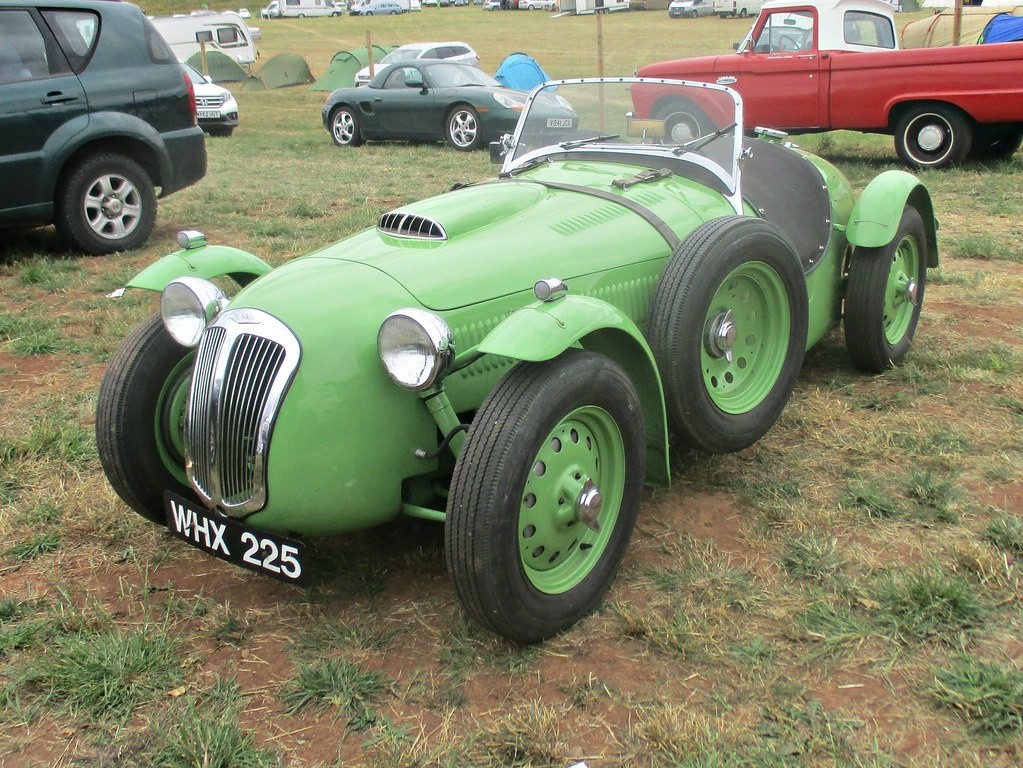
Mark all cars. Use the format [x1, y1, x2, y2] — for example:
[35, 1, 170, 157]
[321, 58, 581, 152]
[360, 2, 403, 16]
[518, 0, 556, 10]
[482, 0, 501, 11]
[0, 0, 208, 259]
[239, 8, 251, 20]
[95, 77, 941, 651]
[352, 41, 482, 87]
[421, 0, 469, 8]
[474, 0, 482, 6]
[178, 63, 240, 138]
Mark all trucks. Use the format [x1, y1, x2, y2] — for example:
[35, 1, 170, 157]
[349, 0, 410, 11]
[261, 0, 280, 19]
[715, 0, 767, 19]
[559, 0, 630, 15]
[278, 0, 343, 19]
[73, 10, 259, 73]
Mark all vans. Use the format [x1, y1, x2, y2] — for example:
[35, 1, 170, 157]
[667, 0, 719, 18]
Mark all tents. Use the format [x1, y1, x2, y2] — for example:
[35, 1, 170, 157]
[492, 53, 558, 92]
[900, 4, 1023, 50]
[309, 46, 401, 91]
[187, 51, 249, 83]
[241, 54, 315, 90]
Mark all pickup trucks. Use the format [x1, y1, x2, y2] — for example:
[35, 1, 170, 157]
[623, 0, 1022, 172]
